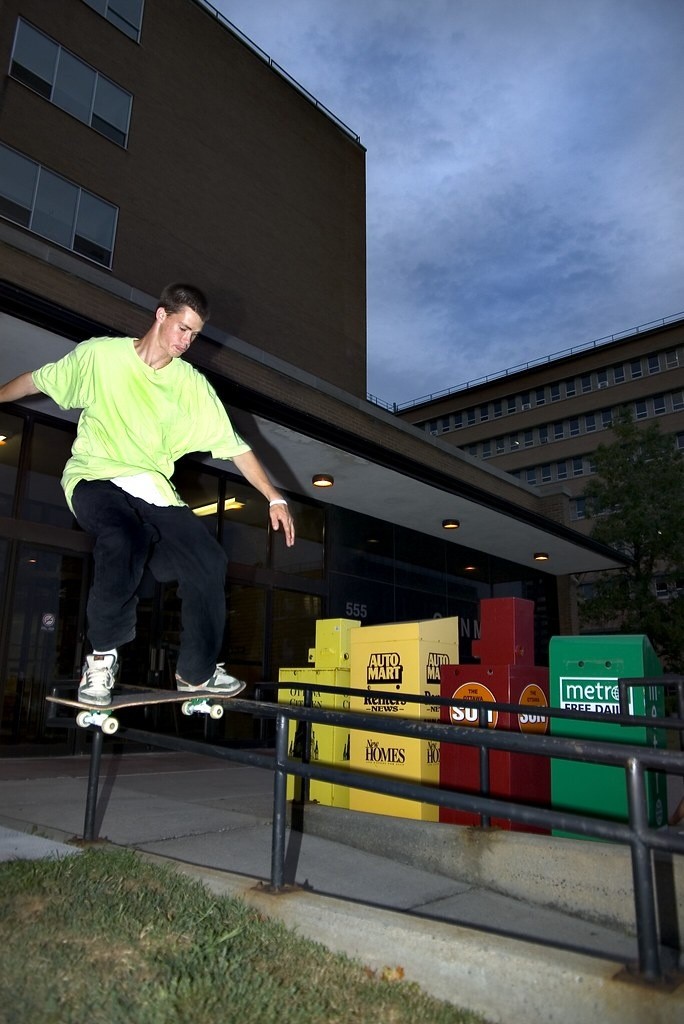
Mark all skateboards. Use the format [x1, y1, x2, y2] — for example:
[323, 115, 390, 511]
[39, 680, 251, 737]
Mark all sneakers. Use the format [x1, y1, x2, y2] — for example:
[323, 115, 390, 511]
[78, 653, 119, 705]
[175, 661, 241, 692]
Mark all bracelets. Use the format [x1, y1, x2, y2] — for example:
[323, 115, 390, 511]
[269, 500, 289, 507]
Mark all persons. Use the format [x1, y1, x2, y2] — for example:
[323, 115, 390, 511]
[1, 282, 296, 707]
